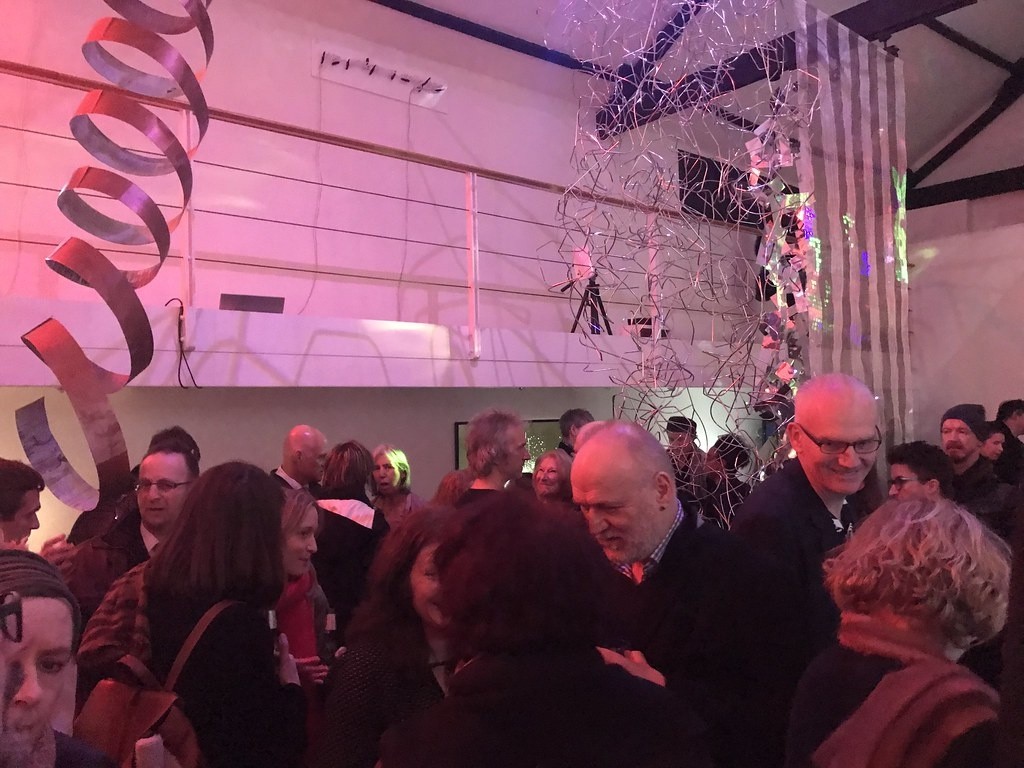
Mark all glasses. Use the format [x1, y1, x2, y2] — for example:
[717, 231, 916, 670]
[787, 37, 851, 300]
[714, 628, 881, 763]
[134, 477, 190, 493]
[888, 476, 919, 489]
[795, 421, 883, 454]
[0, 590, 23, 643]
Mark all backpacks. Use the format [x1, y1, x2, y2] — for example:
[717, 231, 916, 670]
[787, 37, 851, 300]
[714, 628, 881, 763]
[72, 599, 237, 768]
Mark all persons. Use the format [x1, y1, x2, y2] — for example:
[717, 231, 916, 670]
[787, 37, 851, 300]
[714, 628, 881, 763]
[977, 418, 1006, 461]
[992, 397, 1024, 488]
[270, 484, 344, 768]
[733, 371, 885, 768]
[313, 439, 389, 639]
[0, 455, 78, 584]
[39, 425, 202, 651]
[126, 460, 313, 768]
[567, 421, 811, 768]
[366, 445, 427, 531]
[313, 507, 474, 768]
[937, 402, 1013, 690]
[268, 423, 330, 502]
[0, 550, 123, 768]
[886, 439, 957, 503]
[432, 405, 752, 527]
[782, 488, 1024, 768]
[374, 488, 720, 768]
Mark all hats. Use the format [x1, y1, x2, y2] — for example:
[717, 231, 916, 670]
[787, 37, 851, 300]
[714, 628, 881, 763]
[940, 404, 986, 442]
[667, 416, 697, 436]
